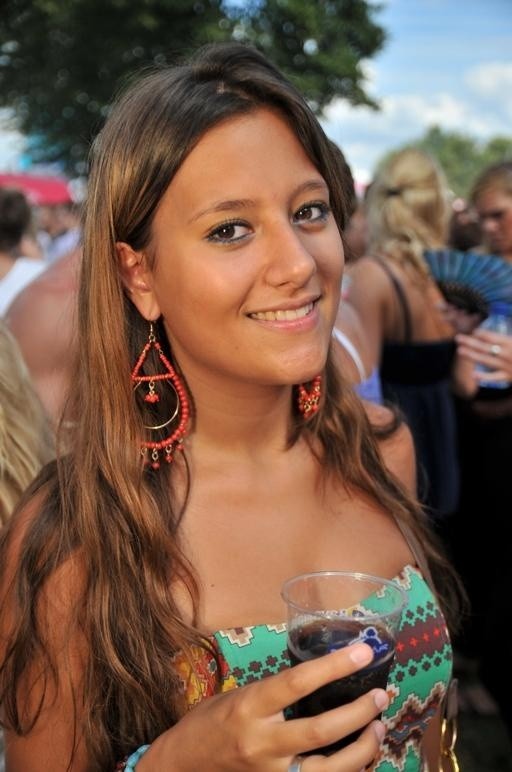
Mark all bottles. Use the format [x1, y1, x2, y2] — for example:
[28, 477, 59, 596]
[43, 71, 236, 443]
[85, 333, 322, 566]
[474, 312, 511, 389]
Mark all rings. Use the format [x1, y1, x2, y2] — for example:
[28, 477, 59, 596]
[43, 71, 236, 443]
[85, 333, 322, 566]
[289, 755, 305, 772]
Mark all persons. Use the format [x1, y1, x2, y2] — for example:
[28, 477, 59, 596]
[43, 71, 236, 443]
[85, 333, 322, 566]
[2, 45, 466, 772]
[330, 147, 512, 772]
[1, 191, 88, 539]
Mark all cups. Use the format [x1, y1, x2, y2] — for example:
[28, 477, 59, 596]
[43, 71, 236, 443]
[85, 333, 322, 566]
[282, 570, 408, 771]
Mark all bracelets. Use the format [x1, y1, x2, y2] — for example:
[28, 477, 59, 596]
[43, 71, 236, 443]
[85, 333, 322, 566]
[115, 744, 148, 772]
